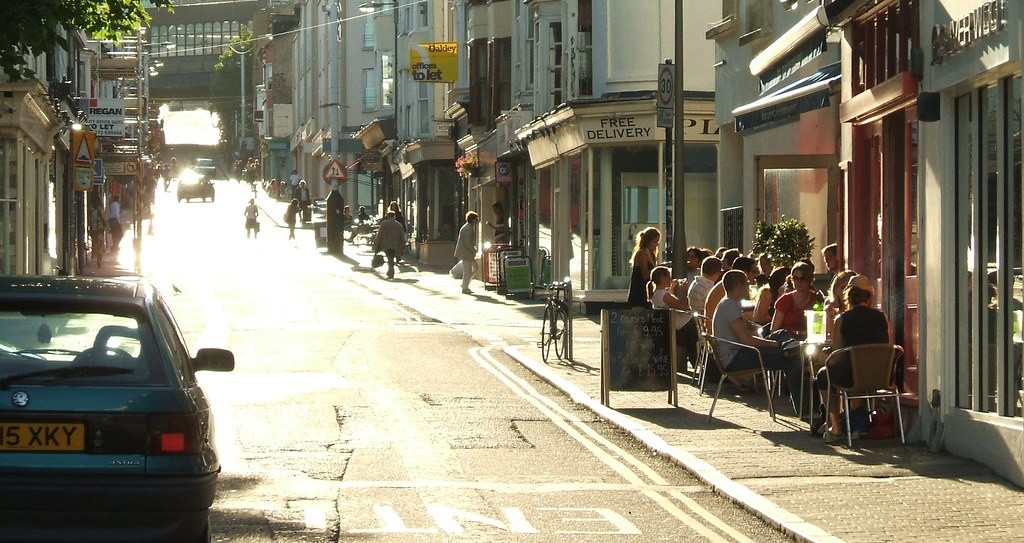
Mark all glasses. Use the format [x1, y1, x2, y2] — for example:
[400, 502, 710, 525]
[790, 275, 809, 282]
[747, 268, 758, 274]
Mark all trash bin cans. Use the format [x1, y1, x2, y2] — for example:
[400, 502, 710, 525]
[315, 220, 326, 248]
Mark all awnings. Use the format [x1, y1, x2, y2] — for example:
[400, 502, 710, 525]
[731, 62, 841, 132]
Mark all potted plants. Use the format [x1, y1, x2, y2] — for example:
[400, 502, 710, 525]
[749, 220, 773, 276]
[769, 218, 829, 295]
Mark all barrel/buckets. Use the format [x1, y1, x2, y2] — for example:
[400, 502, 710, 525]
[807, 311, 827, 343]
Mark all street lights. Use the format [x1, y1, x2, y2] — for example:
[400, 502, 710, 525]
[135, 39, 176, 238]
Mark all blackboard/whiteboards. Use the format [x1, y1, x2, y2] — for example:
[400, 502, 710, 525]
[600, 308, 677, 394]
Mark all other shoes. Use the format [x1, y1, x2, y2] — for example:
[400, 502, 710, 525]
[728, 384, 751, 392]
[783, 340, 804, 356]
[462, 289, 472, 293]
[804, 412, 821, 420]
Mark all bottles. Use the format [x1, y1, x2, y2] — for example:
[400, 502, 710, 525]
[813, 292, 823, 335]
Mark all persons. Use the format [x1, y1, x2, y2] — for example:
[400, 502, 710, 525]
[286, 198, 300, 240]
[235, 157, 302, 202]
[295, 180, 310, 222]
[145, 156, 177, 193]
[244, 199, 258, 238]
[343, 206, 355, 231]
[386, 201, 406, 263]
[357, 206, 369, 224]
[627, 227, 661, 310]
[108, 195, 122, 250]
[454, 211, 479, 294]
[486, 202, 510, 245]
[648, 243, 894, 442]
[966, 268, 1023, 345]
[372, 212, 406, 279]
[91, 206, 105, 252]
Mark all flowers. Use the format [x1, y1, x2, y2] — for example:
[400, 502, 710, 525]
[455, 153, 479, 176]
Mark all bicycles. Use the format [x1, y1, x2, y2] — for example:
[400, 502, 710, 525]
[529, 282, 565, 362]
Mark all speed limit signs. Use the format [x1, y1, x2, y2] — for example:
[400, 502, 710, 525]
[657, 63, 676, 108]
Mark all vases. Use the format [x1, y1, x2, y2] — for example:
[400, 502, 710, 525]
[467, 168, 477, 178]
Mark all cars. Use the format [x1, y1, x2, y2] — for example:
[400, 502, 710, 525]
[177, 174, 214, 204]
[194, 158, 216, 178]
[0, 274, 236, 543]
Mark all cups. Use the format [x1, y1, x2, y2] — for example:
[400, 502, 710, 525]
[749, 285, 758, 302]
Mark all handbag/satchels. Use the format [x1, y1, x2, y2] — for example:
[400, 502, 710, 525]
[371, 255, 385, 267]
[867, 406, 894, 439]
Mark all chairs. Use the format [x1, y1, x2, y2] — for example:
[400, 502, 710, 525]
[669, 307, 906, 447]
[69, 347, 133, 369]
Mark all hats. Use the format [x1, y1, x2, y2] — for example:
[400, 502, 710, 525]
[844, 275, 875, 294]
